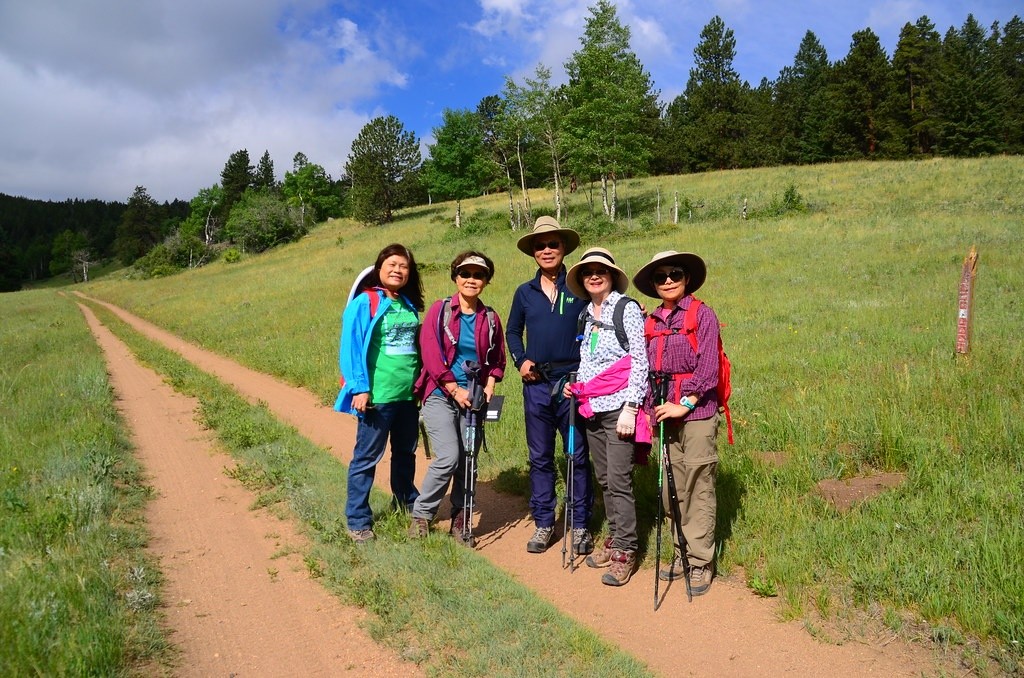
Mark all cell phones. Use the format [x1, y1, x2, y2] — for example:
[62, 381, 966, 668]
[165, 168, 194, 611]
[365, 403, 377, 409]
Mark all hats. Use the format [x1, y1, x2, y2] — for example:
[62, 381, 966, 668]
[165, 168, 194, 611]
[456, 256, 490, 271]
[565, 247, 629, 301]
[632, 250, 707, 300]
[517, 216, 580, 257]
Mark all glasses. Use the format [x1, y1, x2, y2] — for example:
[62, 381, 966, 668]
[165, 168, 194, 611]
[532, 240, 563, 252]
[652, 270, 688, 285]
[580, 268, 609, 276]
[457, 271, 487, 280]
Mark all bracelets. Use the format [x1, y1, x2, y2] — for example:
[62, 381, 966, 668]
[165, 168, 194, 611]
[451, 386, 460, 397]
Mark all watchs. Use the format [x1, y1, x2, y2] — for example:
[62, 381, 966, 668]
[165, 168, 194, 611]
[680, 395, 695, 410]
[625, 402, 640, 409]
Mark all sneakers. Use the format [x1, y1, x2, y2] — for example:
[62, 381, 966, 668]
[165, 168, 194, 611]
[659, 552, 689, 580]
[409, 517, 430, 540]
[602, 549, 636, 586]
[688, 560, 716, 595]
[450, 508, 475, 547]
[586, 538, 616, 569]
[571, 527, 594, 554]
[526, 526, 556, 552]
[349, 528, 377, 547]
[389, 503, 414, 516]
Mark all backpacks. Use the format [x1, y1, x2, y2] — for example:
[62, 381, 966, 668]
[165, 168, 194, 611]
[644, 298, 730, 407]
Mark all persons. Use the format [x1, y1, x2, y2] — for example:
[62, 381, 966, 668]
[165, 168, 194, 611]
[558, 246, 649, 587]
[332, 243, 425, 541]
[632, 250, 722, 597]
[503, 217, 647, 554]
[408, 251, 506, 547]
